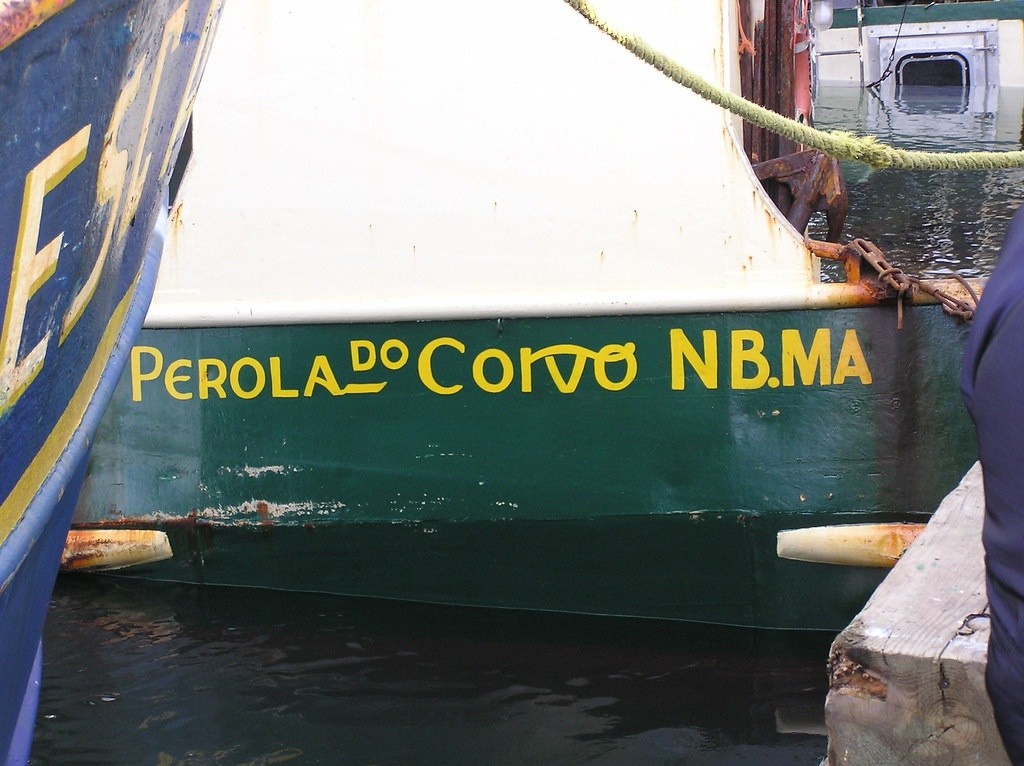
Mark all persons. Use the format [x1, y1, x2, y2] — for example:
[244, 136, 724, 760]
[960, 203, 1024, 766]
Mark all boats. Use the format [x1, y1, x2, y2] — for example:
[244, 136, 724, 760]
[61, 0, 990, 630]
[1, 0, 229, 766]
